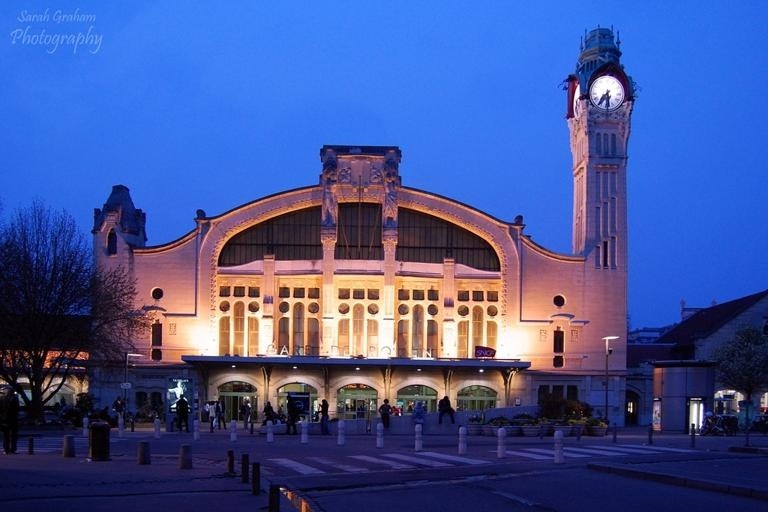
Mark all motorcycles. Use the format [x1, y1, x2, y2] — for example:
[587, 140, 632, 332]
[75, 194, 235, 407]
[699, 414, 736, 436]
[312, 403, 339, 423]
[274, 404, 286, 424]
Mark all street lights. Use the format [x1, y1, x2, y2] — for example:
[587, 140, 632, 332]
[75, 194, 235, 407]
[123, 350, 146, 421]
[601, 336, 620, 434]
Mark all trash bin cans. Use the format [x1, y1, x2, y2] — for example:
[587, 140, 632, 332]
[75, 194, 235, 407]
[87, 421, 110, 461]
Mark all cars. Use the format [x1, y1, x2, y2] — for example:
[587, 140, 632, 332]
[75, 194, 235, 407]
[752, 415, 767, 436]
[56, 402, 164, 430]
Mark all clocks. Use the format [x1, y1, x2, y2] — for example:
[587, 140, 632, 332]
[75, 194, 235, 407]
[590, 75, 625, 111]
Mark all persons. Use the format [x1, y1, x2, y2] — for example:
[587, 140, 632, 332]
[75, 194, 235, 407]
[285, 396, 299, 435]
[75, 397, 79, 409]
[277, 404, 283, 414]
[111, 395, 126, 430]
[438, 396, 455, 425]
[240, 399, 253, 431]
[653, 409, 658, 423]
[317, 403, 323, 422]
[176, 394, 190, 434]
[379, 398, 392, 429]
[60, 396, 66, 408]
[391, 400, 415, 416]
[413, 400, 425, 435]
[655, 413, 660, 423]
[205, 397, 227, 433]
[316, 398, 332, 436]
[0, 384, 21, 456]
[263, 400, 274, 424]
